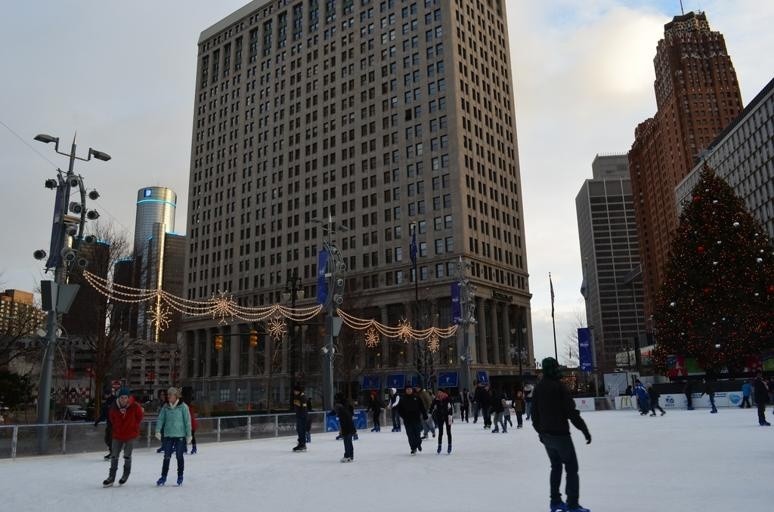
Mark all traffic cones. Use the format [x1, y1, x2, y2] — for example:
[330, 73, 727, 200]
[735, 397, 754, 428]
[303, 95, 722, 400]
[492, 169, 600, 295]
[246, 399, 252, 412]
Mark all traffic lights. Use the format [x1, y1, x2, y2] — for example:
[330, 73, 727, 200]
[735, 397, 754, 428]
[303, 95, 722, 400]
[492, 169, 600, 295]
[211, 333, 223, 351]
[243, 330, 258, 347]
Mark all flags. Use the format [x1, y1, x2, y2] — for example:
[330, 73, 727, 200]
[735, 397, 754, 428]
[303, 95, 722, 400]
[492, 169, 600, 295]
[410, 230, 417, 265]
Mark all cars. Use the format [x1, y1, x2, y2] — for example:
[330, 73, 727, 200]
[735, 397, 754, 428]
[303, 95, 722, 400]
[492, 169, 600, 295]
[54, 403, 88, 420]
[139, 399, 164, 414]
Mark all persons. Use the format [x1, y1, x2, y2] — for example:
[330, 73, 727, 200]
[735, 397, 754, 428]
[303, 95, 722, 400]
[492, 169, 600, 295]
[700, 379, 718, 413]
[86, 385, 143, 486]
[331, 382, 533, 461]
[153, 386, 197, 485]
[530, 357, 591, 512]
[626, 379, 666, 418]
[683, 380, 697, 410]
[738, 374, 774, 426]
[286, 388, 314, 450]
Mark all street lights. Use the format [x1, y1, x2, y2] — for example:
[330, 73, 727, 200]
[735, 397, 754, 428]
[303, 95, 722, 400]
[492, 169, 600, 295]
[309, 209, 350, 430]
[32, 129, 112, 454]
[510, 315, 527, 399]
[619, 338, 633, 372]
[447, 253, 479, 404]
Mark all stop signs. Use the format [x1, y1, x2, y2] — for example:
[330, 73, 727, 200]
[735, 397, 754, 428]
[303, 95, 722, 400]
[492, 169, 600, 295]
[111, 380, 122, 391]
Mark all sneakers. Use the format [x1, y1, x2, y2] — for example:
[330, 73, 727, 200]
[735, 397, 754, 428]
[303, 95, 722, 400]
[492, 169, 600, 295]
[461, 418, 530, 433]
[177, 477, 183, 483]
[157, 477, 166, 484]
[103, 477, 115, 484]
[567, 505, 590, 512]
[410, 432, 452, 454]
[104, 454, 110, 458]
[119, 476, 128, 484]
[334, 433, 359, 464]
[639, 409, 666, 416]
[371, 425, 402, 434]
[293, 434, 312, 451]
[158, 448, 197, 454]
[550, 503, 567, 511]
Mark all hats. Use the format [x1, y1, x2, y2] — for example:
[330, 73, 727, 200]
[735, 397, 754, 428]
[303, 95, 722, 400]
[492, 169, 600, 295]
[542, 357, 560, 370]
[370, 391, 378, 397]
[118, 384, 130, 396]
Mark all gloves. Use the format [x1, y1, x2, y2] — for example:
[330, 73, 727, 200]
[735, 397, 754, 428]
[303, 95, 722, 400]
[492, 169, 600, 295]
[425, 414, 432, 423]
[583, 429, 591, 444]
[155, 433, 161, 440]
[423, 415, 428, 420]
[94, 421, 99, 426]
[447, 415, 453, 426]
[186, 436, 192, 444]
